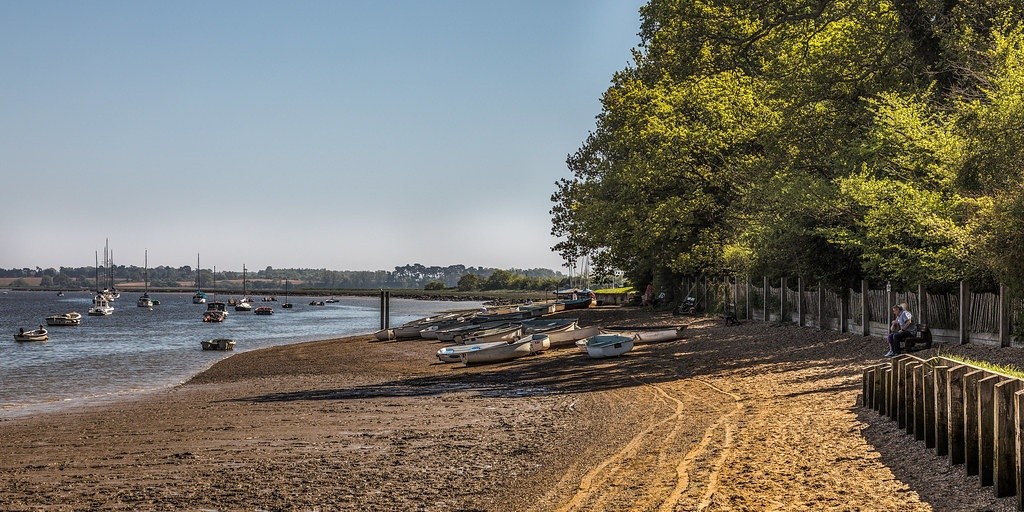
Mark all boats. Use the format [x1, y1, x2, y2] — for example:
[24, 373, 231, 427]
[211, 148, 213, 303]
[200, 337, 237, 351]
[227, 297, 238, 306]
[254, 306, 274, 315]
[151, 299, 161, 305]
[308, 300, 324, 306]
[46, 312, 81, 327]
[597, 323, 689, 344]
[262, 296, 277, 302]
[88, 294, 114, 316]
[325, 297, 340, 303]
[374, 296, 601, 366]
[13, 323, 48, 342]
[574, 334, 634, 358]
[235, 303, 252, 311]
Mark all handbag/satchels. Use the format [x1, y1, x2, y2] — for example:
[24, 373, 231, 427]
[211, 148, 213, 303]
[890, 322, 901, 332]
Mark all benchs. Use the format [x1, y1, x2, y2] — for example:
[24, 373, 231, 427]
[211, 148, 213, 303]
[721, 304, 739, 326]
[906, 324, 932, 350]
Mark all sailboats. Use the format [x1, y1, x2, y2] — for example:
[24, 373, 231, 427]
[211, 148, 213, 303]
[192, 253, 207, 304]
[203, 265, 229, 322]
[282, 279, 292, 308]
[239, 263, 255, 303]
[137, 248, 153, 307]
[91, 238, 121, 305]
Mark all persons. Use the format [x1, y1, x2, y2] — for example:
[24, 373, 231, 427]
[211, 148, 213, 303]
[885, 303, 917, 358]
[524, 299, 533, 304]
[645, 285, 654, 310]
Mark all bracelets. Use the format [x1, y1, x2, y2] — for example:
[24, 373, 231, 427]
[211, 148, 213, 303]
[902, 329, 905, 331]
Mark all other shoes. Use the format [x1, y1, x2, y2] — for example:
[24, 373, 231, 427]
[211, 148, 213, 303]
[889, 353, 902, 357]
[884, 350, 894, 357]
